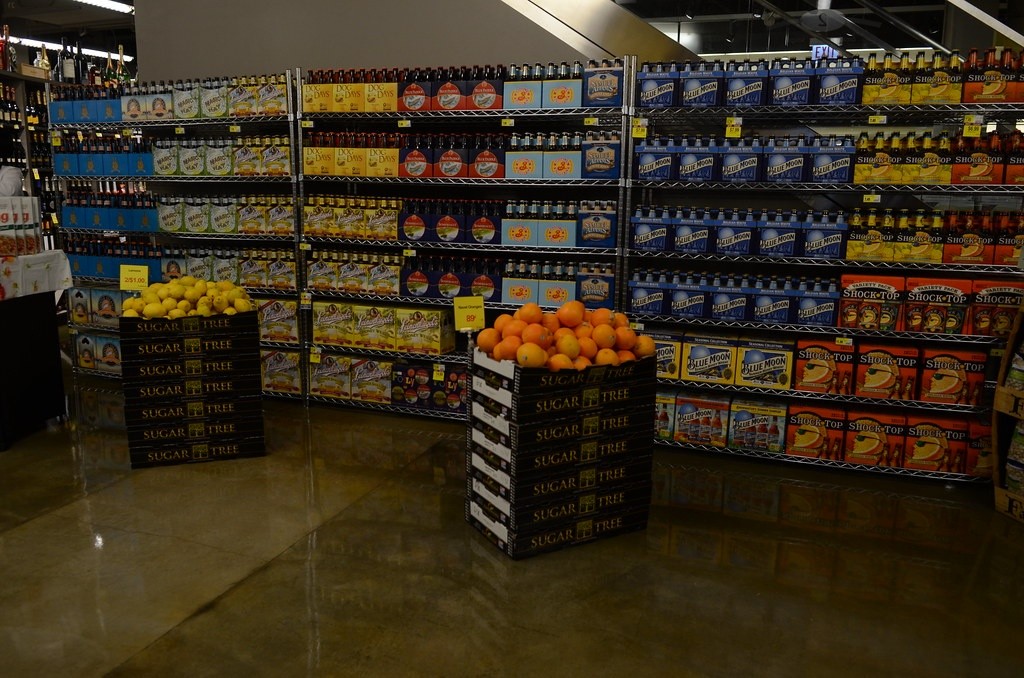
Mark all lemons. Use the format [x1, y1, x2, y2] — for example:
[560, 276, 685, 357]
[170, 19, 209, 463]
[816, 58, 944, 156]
[121, 276, 252, 318]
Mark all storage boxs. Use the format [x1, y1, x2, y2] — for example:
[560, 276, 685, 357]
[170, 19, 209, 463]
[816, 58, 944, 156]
[0, 84, 1024, 559]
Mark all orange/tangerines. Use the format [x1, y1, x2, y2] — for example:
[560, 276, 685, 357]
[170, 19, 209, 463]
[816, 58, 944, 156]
[476, 301, 656, 371]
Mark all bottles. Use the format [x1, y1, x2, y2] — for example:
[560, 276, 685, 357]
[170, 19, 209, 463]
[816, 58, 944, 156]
[826, 370, 851, 393]
[877, 444, 901, 468]
[0, 24, 621, 280]
[955, 382, 981, 405]
[888, 376, 913, 399]
[655, 401, 780, 451]
[935, 449, 964, 474]
[632, 47, 1023, 293]
[816, 437, 840, 462]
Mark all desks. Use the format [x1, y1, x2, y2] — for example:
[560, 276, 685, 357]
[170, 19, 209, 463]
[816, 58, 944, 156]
[1, 248, 73, 452]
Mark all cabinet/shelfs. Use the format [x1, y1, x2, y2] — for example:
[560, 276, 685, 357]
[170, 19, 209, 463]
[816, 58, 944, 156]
[0, 67, 58, 254]
[991, 294, 1024, 527]
[44, 69, 306, 404]
[622, 53, 1024, 485]
[295, 56, 629, 425]
